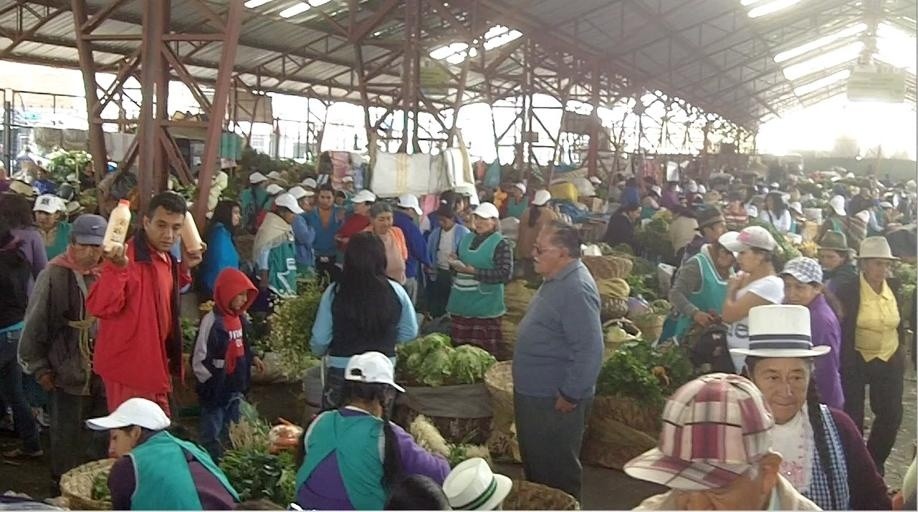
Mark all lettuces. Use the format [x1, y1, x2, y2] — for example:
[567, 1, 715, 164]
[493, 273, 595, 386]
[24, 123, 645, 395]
[394, 334, 496, 389]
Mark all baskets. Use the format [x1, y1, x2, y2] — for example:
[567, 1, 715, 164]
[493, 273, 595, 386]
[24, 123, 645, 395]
[591, 395, 659, 473]
[583, 255, 633, 318]
[59, 457, 116, 510]
[484, 359, 521, 463]
[506, 476, 579, 510]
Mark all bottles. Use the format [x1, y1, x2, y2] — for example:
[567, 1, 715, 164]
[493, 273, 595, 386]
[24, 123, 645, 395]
[180, 209, 204, 255]
[104, 199, 131, 254]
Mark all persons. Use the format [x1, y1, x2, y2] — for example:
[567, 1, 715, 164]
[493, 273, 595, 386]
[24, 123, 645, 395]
[0, 159, 916, 511]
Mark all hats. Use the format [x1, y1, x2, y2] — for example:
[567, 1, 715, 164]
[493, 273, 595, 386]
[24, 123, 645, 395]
[717, 225, 777, 253]
[471, 202, 499, 218]
[762, 181, 802, 214]
[729, 304, 832, 359]
[651, 185, 662, 198]
[776, 257, 823, 285]
[830, 195, 846, 216]
[516, 182, 551, 206]
[72, 212, 107, 244]
[443, 457, 512, 510]
[344, 351, 406, 393]
[590, 176, 602, 184]
[820, 229, 899, 261]
[351, 189, 376, 204]
[623, 371, 775, 491]
[86, 397, 172, 430]
[397, 194, 423, 216]
[251, 170, 317, 214]
[692, 208, 726, 231]
[9, 180, 64, 214]
